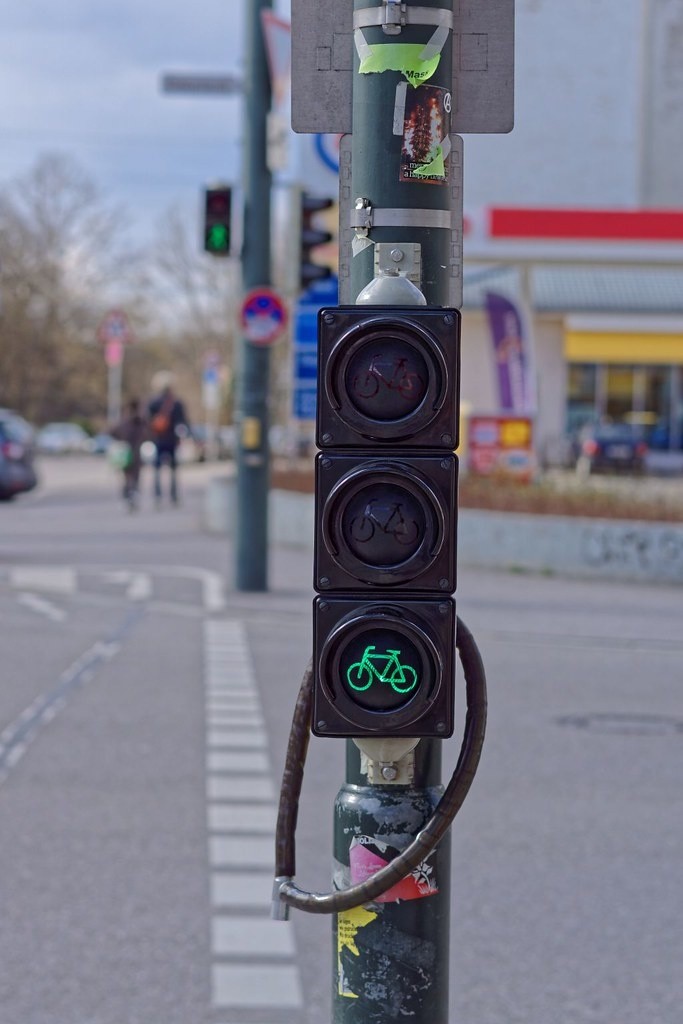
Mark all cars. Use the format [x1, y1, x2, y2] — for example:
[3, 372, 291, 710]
[0, 409, 36, 499]
[575, 423, 647, 476]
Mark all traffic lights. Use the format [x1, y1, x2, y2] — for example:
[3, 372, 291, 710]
[205, 187, 231, 254]
[312, 305, 460, 738]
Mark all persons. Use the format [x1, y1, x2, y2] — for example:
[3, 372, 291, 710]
[566, 413, 614, 475]
[104, 369, 194, 500]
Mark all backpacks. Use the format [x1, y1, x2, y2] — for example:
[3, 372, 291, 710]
[151, 399, 176, 432]
